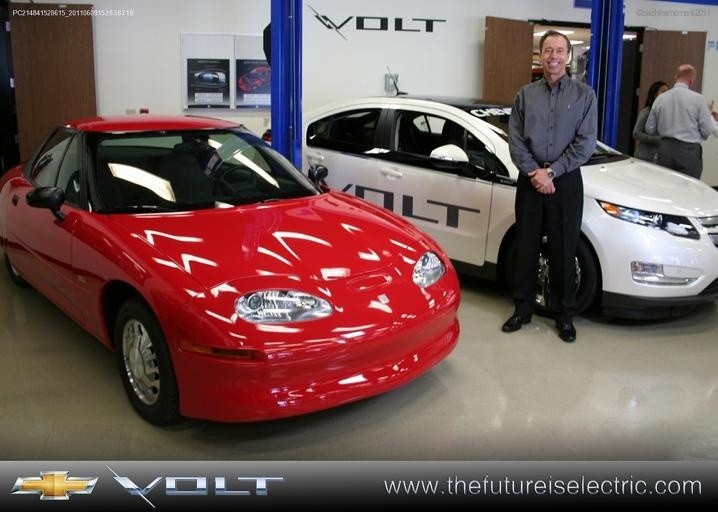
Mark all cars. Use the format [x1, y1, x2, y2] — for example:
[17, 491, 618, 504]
[238, 65, 271, 92]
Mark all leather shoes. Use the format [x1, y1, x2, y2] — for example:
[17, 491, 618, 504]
[555, 315, 576, 341]
[501, 311, 532, 332]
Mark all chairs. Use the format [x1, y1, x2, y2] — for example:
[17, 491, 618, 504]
[158, 141, 220, 202]
[330, 118, 361, 144]
[399, 116, 436, 156]
[437, 120, 468, 162]
[104, 150, 158, 206]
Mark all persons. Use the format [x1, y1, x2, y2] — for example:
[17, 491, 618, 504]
[632, 79, 669, 164]
[645, 64, 717, 181]
[500, 29, 599, 343]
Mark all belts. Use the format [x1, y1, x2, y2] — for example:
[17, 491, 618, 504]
[537, 161, 555, 169]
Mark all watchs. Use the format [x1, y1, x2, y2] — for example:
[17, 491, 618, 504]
[546, 167, 557, 179]
